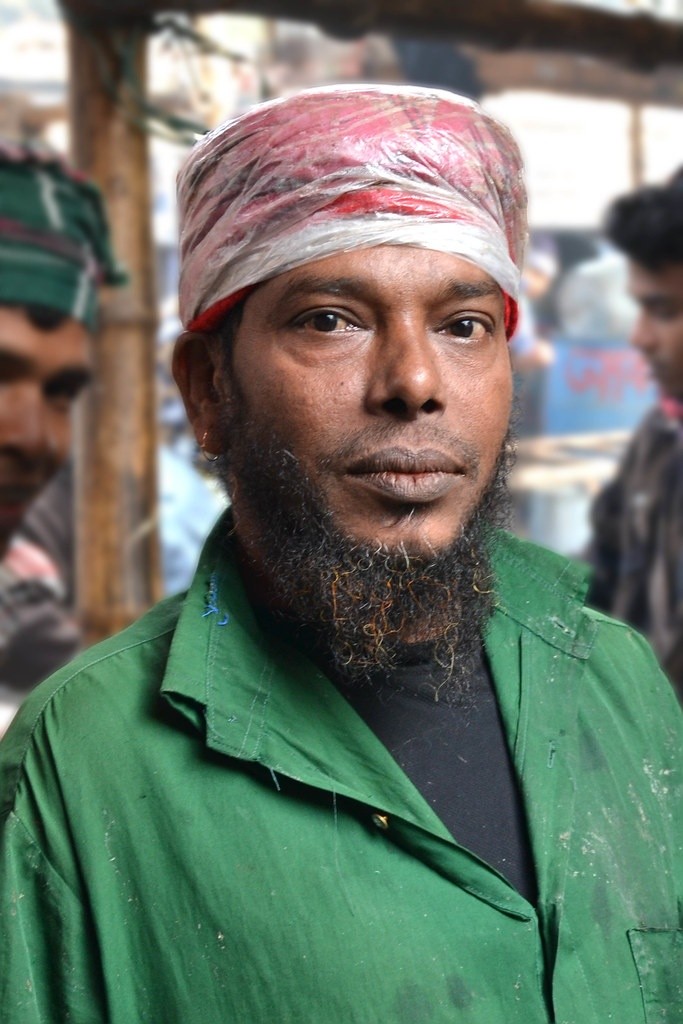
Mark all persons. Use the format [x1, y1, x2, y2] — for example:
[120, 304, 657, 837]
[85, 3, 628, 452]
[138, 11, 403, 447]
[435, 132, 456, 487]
[0, 84, 682, 1024]
[1, 129, 128, 743]
[544, 255, 659, 436]
[509, 249, 560, 366]
[577, 168, 682, 712]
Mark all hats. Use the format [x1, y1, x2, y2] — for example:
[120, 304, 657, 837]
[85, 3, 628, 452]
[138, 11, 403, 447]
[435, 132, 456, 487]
[176, 84, 527, 340]
[0, 141, 129, 330]
